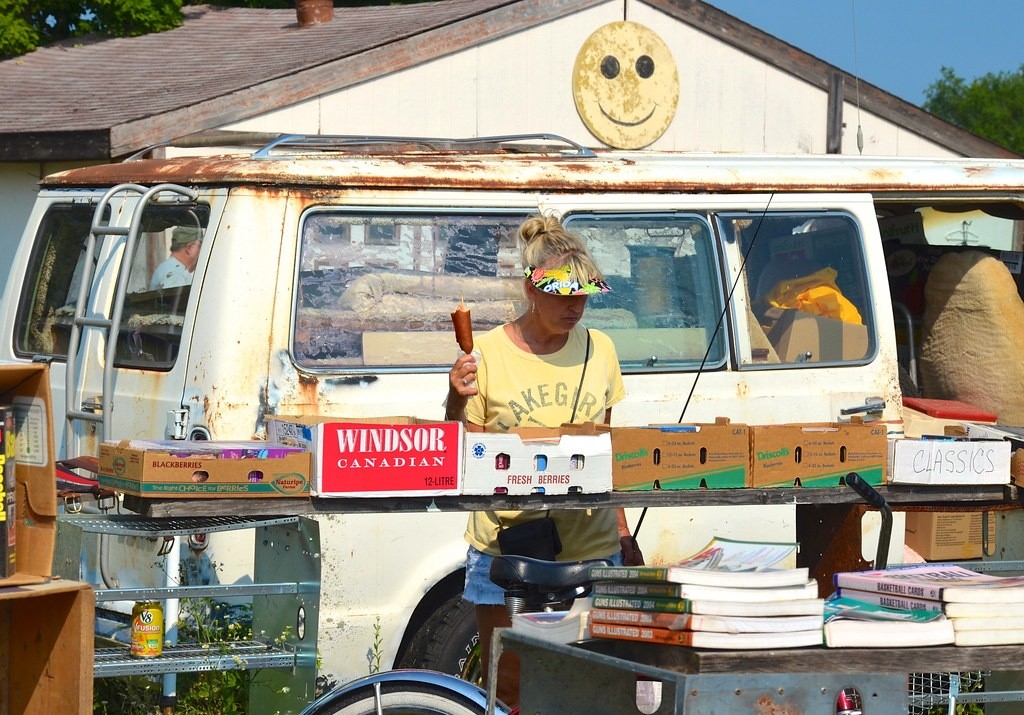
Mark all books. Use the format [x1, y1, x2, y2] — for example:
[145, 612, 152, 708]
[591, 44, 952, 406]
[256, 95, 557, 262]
[512, 536, 1024, 649]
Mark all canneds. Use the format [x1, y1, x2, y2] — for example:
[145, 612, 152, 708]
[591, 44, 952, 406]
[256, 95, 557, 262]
[132, 601, 163, 658]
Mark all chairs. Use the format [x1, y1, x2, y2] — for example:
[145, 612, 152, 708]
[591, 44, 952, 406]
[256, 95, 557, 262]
[920, 252, 1024, 490]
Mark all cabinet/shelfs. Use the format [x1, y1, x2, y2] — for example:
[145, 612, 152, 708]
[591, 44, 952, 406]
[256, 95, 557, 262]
[52, 515, 301, 676]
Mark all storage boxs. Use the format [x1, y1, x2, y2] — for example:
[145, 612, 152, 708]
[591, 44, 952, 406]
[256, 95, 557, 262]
[99, 416, 1014, 498]
[0, 365, 58, 586]
[751, 301, 869, 363]
[903, 510, 995, 560]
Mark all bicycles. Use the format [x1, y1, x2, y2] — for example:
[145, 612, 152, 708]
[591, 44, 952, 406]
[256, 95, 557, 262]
[293, 473, 892, 715]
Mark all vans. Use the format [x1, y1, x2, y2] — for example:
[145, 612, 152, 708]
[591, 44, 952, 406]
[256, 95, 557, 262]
[0, 129, 1024, 691]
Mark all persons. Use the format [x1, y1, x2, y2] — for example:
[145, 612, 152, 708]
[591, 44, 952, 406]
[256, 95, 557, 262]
[443, 214, 646, 706]
[151, 225, 203, 291]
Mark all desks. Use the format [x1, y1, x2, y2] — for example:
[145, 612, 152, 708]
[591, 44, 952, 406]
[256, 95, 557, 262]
[121, 493, 1024, 715]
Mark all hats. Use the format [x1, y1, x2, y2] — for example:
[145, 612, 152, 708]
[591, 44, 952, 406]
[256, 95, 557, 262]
[524, 267, 611, 296]
[171, 226, 203, 244]
